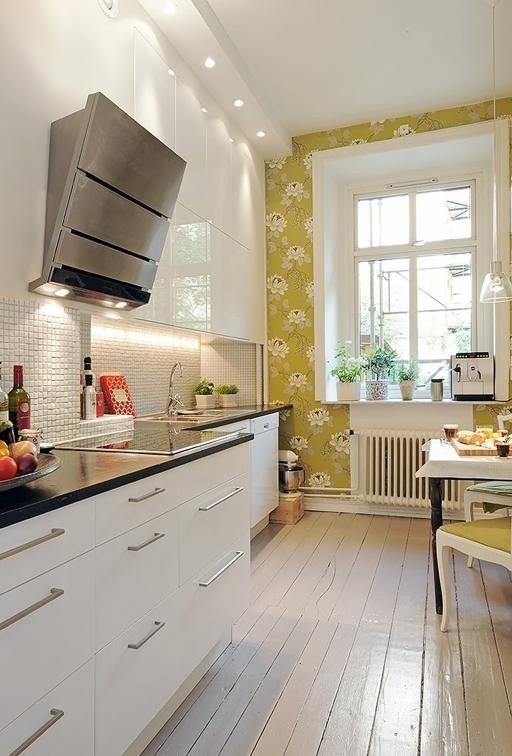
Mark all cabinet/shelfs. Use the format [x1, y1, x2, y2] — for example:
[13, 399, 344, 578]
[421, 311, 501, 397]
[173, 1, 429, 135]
[250, 410, 279, 541]
[200, 418, 250, 435]
[0, 439, 97, 755]
[96, 462, 186, 756]
[182, 443, 255, 696]
[117, 31, 267, 347]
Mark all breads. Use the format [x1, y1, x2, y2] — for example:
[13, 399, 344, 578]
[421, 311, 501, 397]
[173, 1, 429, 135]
[457, 430, 484, 445]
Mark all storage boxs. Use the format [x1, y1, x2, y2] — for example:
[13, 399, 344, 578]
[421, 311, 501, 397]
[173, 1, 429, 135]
[269, 492, 305, 525]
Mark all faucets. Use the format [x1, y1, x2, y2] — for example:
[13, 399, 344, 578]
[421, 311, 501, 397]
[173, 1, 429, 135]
[168, 362, 183, 416]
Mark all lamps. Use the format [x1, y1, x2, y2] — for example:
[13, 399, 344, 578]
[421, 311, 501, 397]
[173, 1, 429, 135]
[479, 2, 512, 304]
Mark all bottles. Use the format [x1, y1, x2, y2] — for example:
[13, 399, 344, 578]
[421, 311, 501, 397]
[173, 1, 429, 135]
[80, 373, 83, 418]
[0, 362, 9, 421]
[8, 365, 31, 441]
[431, 378, 444, 402]
[83, 357, 96, 388]
[83, 375, 97, 420]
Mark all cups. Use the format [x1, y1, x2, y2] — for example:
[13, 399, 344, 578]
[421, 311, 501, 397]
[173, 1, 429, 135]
[495, 442, 510, 457]
[15, 429, 40, 455]
[95, 392, 104, 416]
[441, 424, 459, 441]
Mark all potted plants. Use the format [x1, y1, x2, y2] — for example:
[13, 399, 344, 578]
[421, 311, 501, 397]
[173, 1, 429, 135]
[396, 364, 418, 400]
[214, 384, 240, 408]
[328, 343, 366, 402]
[364, 347, 399, 400]
[192, 377, 216, 408]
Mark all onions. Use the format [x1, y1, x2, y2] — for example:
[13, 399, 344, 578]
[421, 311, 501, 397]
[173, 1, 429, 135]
[14, 453, 38, 475]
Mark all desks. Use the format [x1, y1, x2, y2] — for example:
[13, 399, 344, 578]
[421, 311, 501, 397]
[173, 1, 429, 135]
[415, 438, 511, 615]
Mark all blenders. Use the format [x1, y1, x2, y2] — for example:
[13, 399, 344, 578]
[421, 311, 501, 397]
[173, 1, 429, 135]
[279, 450, 304, 497]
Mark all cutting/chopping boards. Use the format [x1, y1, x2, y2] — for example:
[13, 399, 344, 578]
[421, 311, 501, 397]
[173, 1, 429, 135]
[451, 439, 512, 457]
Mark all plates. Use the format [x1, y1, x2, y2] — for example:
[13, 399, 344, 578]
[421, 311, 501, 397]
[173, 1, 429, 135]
[0, 452, 63, 492]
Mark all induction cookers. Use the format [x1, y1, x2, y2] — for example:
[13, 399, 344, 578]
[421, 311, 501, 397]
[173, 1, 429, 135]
[64, 426, 247, 454]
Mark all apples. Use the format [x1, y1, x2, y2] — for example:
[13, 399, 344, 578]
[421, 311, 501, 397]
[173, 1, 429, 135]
[0, 456, 17, 481]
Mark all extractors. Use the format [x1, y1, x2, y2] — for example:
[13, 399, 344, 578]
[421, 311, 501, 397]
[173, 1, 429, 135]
[29, 92, 188, 312]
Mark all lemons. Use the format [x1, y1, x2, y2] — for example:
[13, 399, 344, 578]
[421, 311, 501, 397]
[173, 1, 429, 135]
[0, 449, 10, 459]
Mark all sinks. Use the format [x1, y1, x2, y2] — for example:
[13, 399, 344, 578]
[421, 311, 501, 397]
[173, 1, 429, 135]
[178, 409, 222, 416]
[154, 417, 201, 421]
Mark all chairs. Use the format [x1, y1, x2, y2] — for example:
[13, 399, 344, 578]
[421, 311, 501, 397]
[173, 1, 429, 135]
[464, 479, 512, 568]
[435, 517, 512, 632]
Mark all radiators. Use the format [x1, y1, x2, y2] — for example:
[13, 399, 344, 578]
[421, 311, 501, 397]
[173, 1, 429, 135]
[357, 428, 463, 511]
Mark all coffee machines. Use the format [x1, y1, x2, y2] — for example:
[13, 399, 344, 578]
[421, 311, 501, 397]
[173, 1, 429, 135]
[450, 351, 495, 400]
